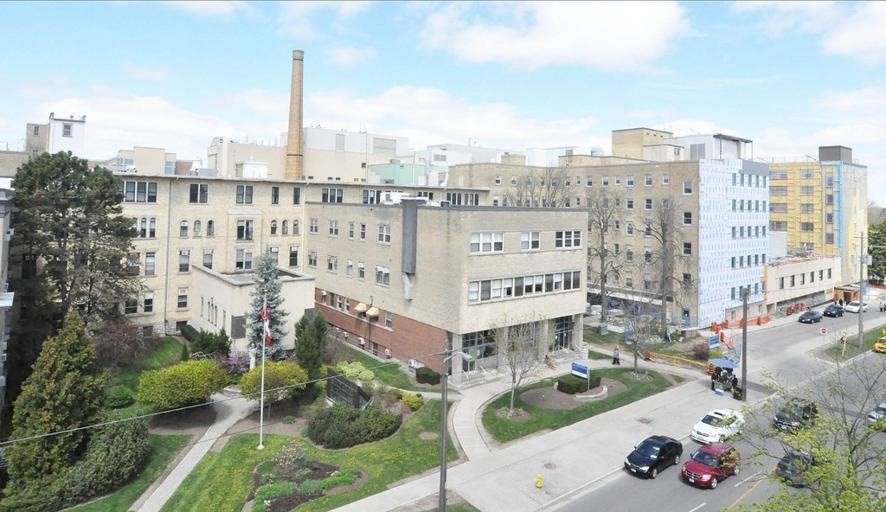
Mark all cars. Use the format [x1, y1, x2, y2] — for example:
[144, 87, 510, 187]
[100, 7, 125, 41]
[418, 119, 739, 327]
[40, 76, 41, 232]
[824, 304, 844, 318]
[689, 408, 744, 444]
[772, 398, 818, 434]
[866, 402, 886, 431]
[874, 336, 886, 353]
[625, 434, 682, 479]
[798, 310, 822, 324]
[846, 299, 866, 313]
[681, 442, 742, 488]
[776, 448, 832, 487]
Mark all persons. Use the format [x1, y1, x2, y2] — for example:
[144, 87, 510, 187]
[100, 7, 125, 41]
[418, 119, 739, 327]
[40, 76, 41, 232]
[612, 345, 620, 366]
[806, 307, 810, 312]
[832, 298, 849, 307]
[883, 303, 886, 312]
[879, 301, 884, 312]
[545, 355, 555, 368]
[709, 364, 738, 391]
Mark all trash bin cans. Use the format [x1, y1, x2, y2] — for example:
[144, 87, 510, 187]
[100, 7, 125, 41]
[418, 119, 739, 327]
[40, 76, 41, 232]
[734, 386, 742, 400]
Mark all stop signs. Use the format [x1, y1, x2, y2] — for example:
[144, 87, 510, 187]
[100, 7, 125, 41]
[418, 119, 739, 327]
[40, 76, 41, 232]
[820, 328, 827, 334]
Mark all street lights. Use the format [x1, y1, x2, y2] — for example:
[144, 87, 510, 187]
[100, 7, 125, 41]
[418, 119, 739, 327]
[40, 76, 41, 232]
[439, 348, 473, 512]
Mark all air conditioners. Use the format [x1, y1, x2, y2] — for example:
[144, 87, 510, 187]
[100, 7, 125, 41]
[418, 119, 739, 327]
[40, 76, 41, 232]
[320, 291, 326, 295]
[336, 328, 364, 344]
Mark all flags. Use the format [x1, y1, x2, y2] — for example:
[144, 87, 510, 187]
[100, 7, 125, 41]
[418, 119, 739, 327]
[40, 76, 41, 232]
[261, 302, 274, 346]
